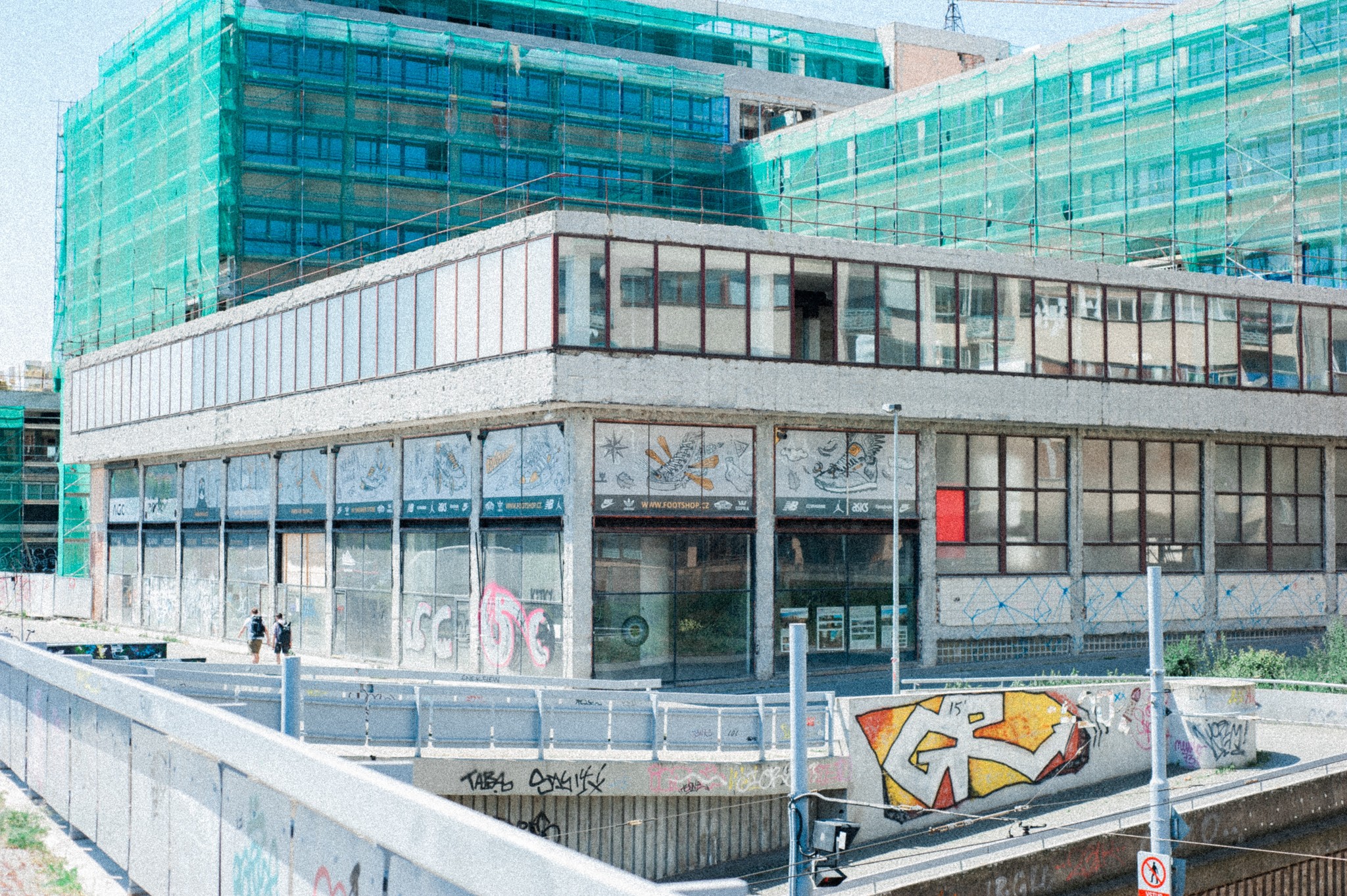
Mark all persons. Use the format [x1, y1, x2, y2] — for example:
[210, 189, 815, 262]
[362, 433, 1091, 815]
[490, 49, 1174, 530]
[273, 613, 293, 664]
[239, 608, 270, 664]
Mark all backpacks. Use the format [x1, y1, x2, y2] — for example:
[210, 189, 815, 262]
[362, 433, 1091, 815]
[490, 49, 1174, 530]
[276, 622, 292, 644]
[252, 616, 265, 638]
